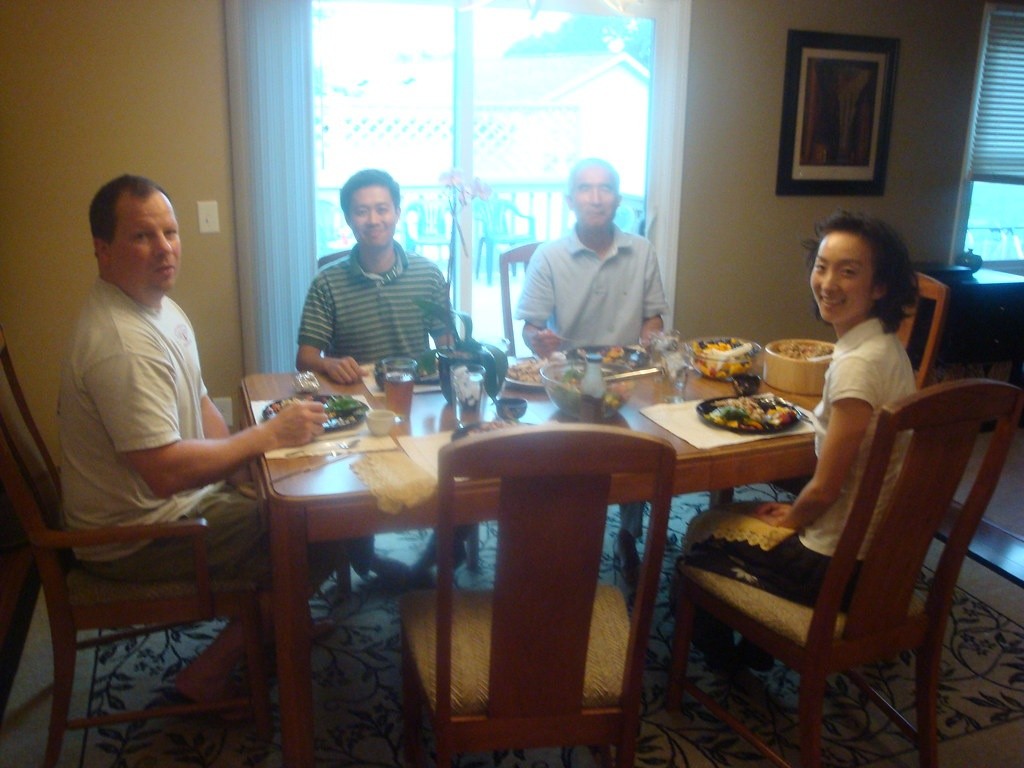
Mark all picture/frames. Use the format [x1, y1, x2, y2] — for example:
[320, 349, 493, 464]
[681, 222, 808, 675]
[774, 30, 902, 194]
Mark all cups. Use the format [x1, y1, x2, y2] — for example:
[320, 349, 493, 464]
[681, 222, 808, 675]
[450, 364, 486, 430]
[366, 411, 395, 435]
[382, 357, 417, 416]
[496, 398, 527, 420]
[645, 366, 689, 405]
[732, 374, 759, 395]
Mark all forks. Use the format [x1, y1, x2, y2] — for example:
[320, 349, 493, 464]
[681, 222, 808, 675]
[286, 440, 359, 458]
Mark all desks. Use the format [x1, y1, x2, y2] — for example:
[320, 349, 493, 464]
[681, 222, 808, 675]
[237, 351, 844, 767]
[877, 264, 1024, 430]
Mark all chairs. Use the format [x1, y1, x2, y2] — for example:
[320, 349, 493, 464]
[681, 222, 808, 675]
[315, 253, 480, 587]
[675, 378, 1024, 768]
[499, 238, 546, 368]
[0, 330, 270, 768]
[399, 422, 678, 767]
[708, 272, 952, 507]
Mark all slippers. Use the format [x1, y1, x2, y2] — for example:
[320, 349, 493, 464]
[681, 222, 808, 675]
[159, 687, 256, 727]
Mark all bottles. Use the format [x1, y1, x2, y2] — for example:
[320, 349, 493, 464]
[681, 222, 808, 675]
[580, 354, 606, 424]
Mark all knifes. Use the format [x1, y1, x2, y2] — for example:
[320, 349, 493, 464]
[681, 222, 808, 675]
[271, 455, 347, 483]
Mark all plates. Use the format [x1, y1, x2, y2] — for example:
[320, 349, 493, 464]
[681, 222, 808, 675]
[504, 376, 544, 387]
[375, 364, 440, 385]
[262, 395, 369, 430]
[565, 346, 650, 373]
[452, 422, 536, 443]
[696, 395, 801, 434]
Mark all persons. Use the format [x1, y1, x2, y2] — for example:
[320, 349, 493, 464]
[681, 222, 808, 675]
[295, 168, 457, 582]
[681, 206, 921, 687]
[514, 156, 668, 584]
[53, 175, 328, 722]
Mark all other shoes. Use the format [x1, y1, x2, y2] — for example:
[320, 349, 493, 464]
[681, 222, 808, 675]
[735, 644, 776, 673]
[703, 652, 749, 677]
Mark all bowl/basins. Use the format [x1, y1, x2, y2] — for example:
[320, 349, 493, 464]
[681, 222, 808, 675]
[763, 338, 836, 394]
[686, 338, 761, 380]
[540, 359, 634, 420]
